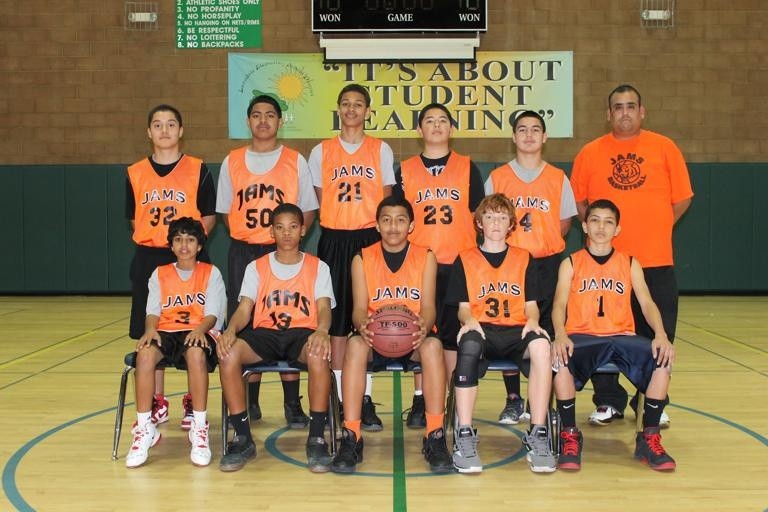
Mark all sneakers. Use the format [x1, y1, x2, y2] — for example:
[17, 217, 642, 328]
[635, 406, 671, 428]
[406, 393, 427, 430]
[498, 392, 525, 425]
[587, 403, 625, 425]
[180, 393, 196, 431]
[324, 401, 346, 431]
[306, 427, 364, 475]
[422, 424, 482, 477]
[360, 393, 382, 435]
[131, 393, 170, 435]
[284, 398, 309, 430]
[525, 396, 561, 425]
[225, 404, 263, 430]
[218, 434, 258, 472]
[634, 425, 678, 472]
[522, 423, 584, 474]
[124, 419, 162, 470]
[188, 418, 212, 466]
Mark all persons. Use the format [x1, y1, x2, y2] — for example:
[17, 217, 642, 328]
[216, 202, 338, 475]
[572, 84, 696, 432]
[483, 109, 580, 425]
[548, 197, 679, 472]
[126, 101, 214, 431]
[121, 215, 228, 470]
[215, 92, 318, 430]
[308, 82, 397, 432]
[440, 193, 556, 476]
[327, 193, 457, 475]
[392, 98, 485, 430]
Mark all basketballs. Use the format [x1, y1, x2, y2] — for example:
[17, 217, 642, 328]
[367, 304, 421, 358]
